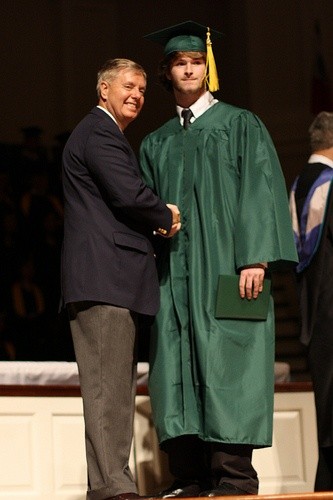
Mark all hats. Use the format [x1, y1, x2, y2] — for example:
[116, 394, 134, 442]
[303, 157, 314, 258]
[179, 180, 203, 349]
[143, 20, 227, 93]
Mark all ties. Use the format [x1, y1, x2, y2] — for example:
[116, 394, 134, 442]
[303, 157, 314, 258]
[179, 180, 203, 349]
[181, 109, 194, 130]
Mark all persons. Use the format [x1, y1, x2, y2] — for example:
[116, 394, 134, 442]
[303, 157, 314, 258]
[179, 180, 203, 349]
[63, 56, 182, 500]
[0, 161, 63, 361]
[137, 18, 299, 499]
[288, 112, 333, 492]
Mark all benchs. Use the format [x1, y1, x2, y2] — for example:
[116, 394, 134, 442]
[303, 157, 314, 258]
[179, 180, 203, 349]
[0, 382, 319, 500]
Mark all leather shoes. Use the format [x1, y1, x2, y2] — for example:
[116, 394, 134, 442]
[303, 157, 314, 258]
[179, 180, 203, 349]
[197, 483, 254, 497]
[106, 492, 145, 500]
[158, 480, 207, 499]
